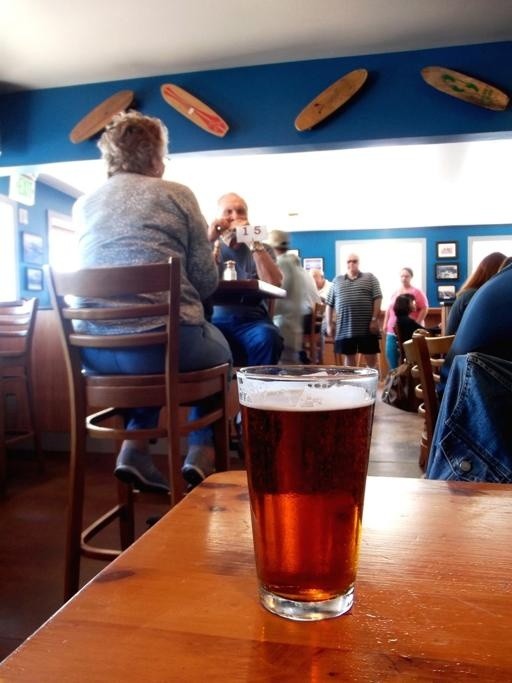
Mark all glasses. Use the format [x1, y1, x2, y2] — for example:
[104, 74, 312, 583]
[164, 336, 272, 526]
[347, 260, 360, 263]
[163, 152, 171, 162]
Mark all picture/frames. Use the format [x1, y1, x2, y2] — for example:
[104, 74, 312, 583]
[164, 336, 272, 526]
[437, 285, 456, 300]
[437, 242, 457, 259]
[436, 263, 459, 280]
[304, 258, 323, 275]
[26, 269, 43, 291]
[23, 232, 44, 264]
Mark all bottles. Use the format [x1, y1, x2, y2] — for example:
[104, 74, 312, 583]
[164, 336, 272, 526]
[223, 260, 237, 280]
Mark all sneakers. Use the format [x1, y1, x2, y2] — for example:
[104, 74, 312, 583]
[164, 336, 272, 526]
[114, 448, 173, 492]
[181, 451, 213, 484]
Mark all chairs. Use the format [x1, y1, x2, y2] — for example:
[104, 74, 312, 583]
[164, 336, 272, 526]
[0, 297, 47, 476]
[392, 324, 404, 366]
[399, 328, 456, 474]
[49, 256, 230, 601]
[312, 303, 324, 365]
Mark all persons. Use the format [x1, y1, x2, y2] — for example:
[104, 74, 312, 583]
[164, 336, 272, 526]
[206, 193, 285, 366]
[325, 252, 382, 369]
[383, 268, 428, 372]
[444, 252, 509, 338]
[394, 294, 441, 359]
[261, 231, 301, 363]
[309, 269, 334, 337]
[424, 264, 512, 484]
[287, 255, 321, 364]
[65, 113, 232, 495]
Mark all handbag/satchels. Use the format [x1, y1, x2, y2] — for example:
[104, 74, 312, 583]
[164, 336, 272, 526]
[381, 358, 426, 413]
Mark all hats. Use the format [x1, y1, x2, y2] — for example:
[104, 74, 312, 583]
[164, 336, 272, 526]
[264, 230, 288, 248]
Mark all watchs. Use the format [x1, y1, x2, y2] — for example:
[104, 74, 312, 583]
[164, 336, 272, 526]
[371, 317, 378, 321]
[246, 242, 267, 253]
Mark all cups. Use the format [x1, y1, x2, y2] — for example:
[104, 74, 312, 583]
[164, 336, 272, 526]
[238, 366, 378, 622]
